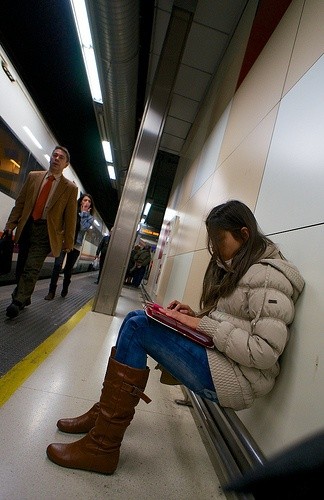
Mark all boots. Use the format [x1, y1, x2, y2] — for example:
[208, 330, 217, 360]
[57, 345, 119, 434]
[45, 359, 152, 475]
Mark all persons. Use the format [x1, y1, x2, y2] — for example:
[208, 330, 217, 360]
[94, 226, 114, 284]
[5, 146, 78, 318]
[46, 200, 305, 476]
[123, 245, 151, 289]
[45, 194, 94, 301]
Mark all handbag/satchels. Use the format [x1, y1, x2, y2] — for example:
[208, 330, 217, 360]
[93, 258, 98, 268]
[0, 230, 13, 274]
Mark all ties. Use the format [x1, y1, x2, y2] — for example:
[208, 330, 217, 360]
[32, 175, 56, 222]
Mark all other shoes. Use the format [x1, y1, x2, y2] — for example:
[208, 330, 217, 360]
[94, 281, 98, 284]
[19, 300, 32, 310]
[45, 292, 54, 300]
[61, 285, 69, 298]
[6, 303, 20, 318]
